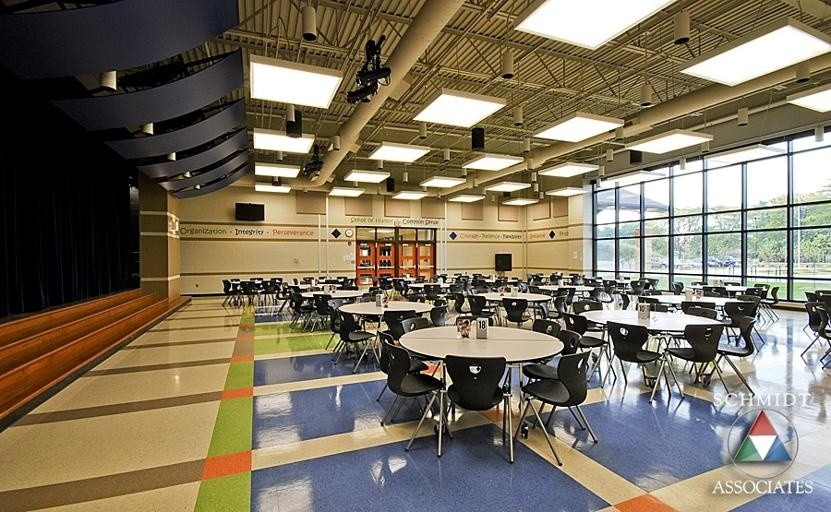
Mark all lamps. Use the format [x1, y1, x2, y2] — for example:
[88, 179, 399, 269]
[100, 71, 117, 92]
[142, 122, 154, 136]
[273, 7, 824, 200]
[195, 184, 200, 189]
[183, 171, 192, 178]
[168, 151, 176, 161]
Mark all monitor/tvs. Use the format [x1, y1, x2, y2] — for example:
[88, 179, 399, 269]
[235, 203, 264, 221]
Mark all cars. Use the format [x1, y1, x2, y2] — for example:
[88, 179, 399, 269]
[650, 256, 735, 269]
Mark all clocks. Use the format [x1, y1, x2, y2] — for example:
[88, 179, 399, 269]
[345, 229, 353, 237]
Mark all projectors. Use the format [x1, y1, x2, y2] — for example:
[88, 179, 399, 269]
[303, 161, 324, 174]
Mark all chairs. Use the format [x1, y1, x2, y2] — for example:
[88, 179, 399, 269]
[221, 273, 831, 466]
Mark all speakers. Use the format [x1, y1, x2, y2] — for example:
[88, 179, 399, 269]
[495, 254, 511, 271]
[472, 128, 484, 151]
[503, 192, 511, 197]
[630, 151, 642, 165]
[286, 110, 302, 138]
[387, 178, 394, 192]
[272, 177, 281, 186]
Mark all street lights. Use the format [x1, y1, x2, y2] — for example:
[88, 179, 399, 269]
[795, 191, 805, 265]
